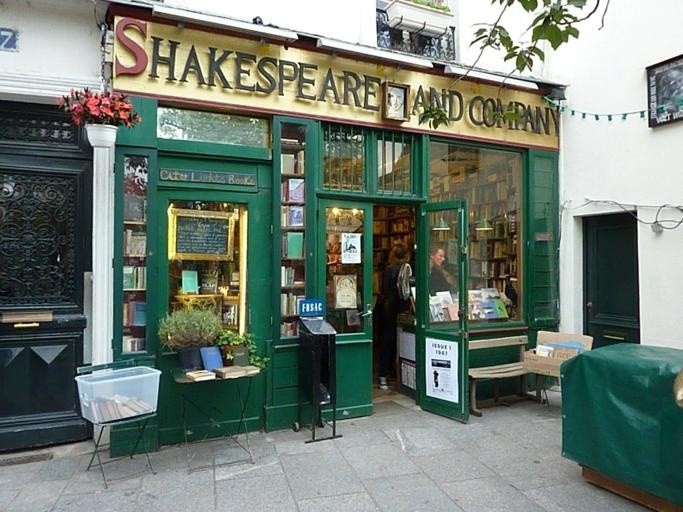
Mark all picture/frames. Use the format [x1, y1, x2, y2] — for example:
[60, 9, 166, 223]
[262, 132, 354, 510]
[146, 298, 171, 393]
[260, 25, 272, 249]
[167, 208, 235, 262]
[644, 54, 682, 128]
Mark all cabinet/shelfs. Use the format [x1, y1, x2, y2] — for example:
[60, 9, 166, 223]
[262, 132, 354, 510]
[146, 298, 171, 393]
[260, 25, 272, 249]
[121, 220, 147, 327]
[373, 160, 517, 312]
[280, 142, 305, 318]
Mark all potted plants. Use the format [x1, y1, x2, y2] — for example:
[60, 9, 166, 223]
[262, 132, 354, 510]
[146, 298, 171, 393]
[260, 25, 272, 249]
[158, 308, 271, 370]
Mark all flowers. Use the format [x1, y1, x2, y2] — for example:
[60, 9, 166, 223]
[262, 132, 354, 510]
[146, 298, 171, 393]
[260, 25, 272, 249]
[55, 86, 143, 131]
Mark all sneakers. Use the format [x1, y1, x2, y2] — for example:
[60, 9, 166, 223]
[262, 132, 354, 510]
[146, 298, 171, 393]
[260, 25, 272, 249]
[377, 375, 389, 391]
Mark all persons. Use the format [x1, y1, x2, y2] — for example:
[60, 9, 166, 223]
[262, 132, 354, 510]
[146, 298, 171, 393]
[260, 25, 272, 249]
[375, 243, 412, 390]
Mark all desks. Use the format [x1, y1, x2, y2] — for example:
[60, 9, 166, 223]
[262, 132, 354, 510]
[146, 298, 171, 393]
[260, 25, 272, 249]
[79, 413, 158, 489]
[170, 369, 261, 474]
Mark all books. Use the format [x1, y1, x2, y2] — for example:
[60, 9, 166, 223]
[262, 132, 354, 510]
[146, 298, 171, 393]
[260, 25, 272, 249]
[533, 340, 582, 360]
[80, 394, 151, 422]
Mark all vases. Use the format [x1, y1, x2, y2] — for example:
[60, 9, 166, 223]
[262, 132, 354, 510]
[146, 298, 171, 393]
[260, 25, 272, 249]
[82, 123, 118, 149]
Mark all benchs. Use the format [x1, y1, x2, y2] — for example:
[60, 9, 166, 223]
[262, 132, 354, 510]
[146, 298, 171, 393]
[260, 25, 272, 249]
[468, 335, 546, 417]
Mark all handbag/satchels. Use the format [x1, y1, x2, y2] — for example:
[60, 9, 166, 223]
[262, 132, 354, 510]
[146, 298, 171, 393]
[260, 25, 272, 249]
[395, 261, 412, 301]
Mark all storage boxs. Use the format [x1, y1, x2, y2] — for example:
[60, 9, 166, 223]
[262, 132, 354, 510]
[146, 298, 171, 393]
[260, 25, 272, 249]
[73, 366, 162, 424]
